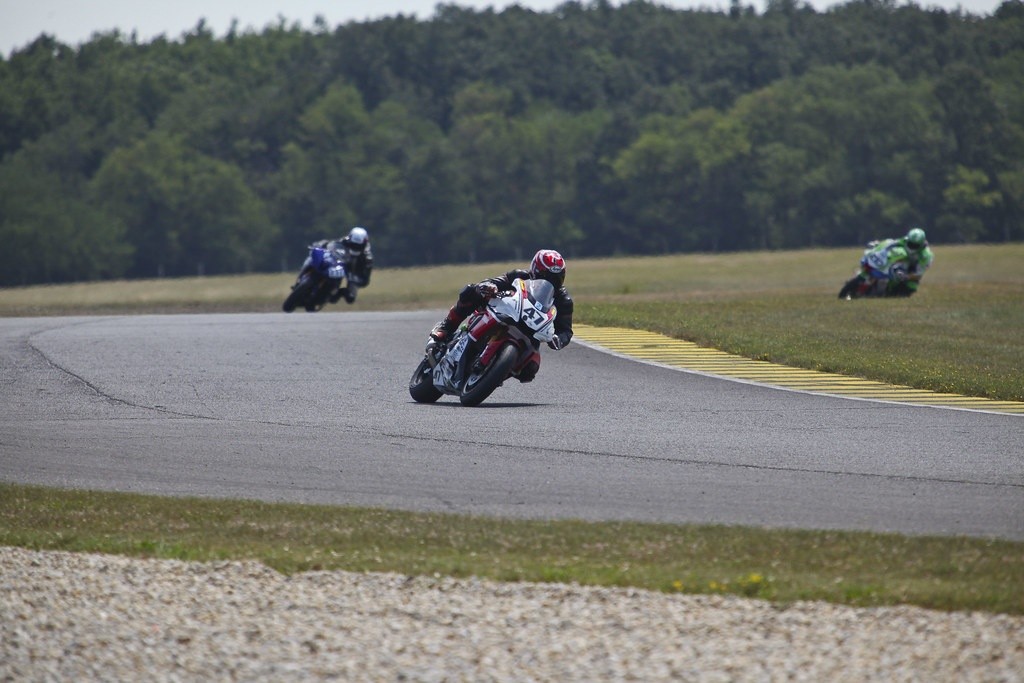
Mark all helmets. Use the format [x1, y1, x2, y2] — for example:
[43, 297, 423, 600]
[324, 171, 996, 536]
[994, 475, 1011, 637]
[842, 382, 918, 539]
[905, 229, 926, 254]
[347, 227, 368, 256]
[530, 250, 565, 290]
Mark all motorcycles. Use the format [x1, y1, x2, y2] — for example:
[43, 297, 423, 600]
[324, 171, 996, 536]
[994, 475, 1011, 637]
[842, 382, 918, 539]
[281, 235, 351, 313]
[407, 278, 560, 408]
[838, 237, 911, 301]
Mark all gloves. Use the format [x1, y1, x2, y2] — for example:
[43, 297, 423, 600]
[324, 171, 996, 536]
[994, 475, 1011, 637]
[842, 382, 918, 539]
[867, 241, 876, 249]
[479, 281, 498, 297]
[896, 271, 904, 282]
[547, 335, 560, 350]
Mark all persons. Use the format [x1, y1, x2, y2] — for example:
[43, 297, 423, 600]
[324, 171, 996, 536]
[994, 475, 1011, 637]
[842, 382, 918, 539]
[292, 228, 372, 304]
[861, 229, 934, 298]
[431, 250, 574, 388]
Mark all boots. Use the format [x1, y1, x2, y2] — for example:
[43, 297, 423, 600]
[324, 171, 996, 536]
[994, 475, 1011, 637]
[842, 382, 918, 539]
[433, 306, 465, 340]
